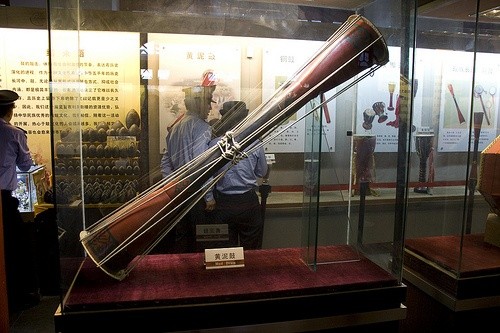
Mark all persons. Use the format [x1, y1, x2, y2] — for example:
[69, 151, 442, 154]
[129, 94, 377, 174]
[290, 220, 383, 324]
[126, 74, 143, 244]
[0, 90, 40, 314]
[207, 100, 268, 251]
[161, 85, 217, 253]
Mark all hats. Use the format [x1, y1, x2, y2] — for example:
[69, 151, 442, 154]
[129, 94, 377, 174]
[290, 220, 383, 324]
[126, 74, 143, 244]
[219, 101, 249, 119]
[0, 90, 18, 107]
[182, 85, 216, 104]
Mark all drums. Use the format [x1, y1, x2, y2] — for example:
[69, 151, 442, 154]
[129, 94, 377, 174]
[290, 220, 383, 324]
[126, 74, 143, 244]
[76, 12, 393, 284]
[465, 111, 485, 234]
[373, 101, 389, 123]
[413, 134, 435, 193]
[361, 108, 376, 130]
[349, 134, 377, 195]
[446, 83, 466, 124]
[387, 83, 395, 111]
